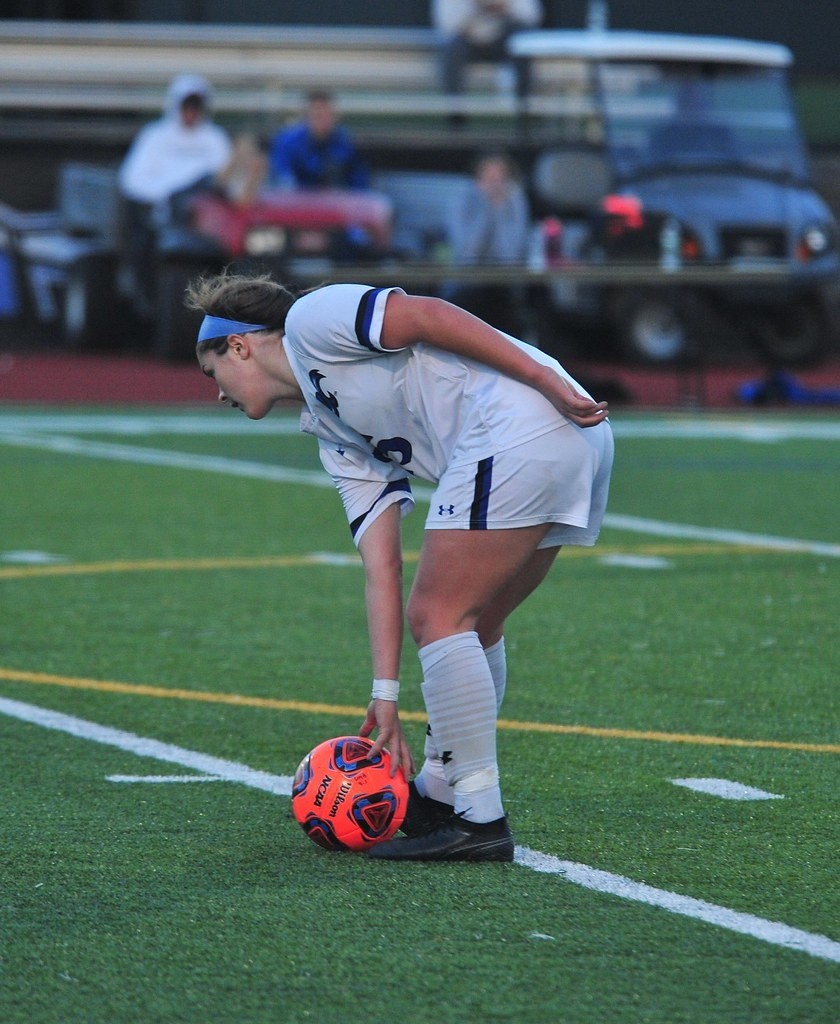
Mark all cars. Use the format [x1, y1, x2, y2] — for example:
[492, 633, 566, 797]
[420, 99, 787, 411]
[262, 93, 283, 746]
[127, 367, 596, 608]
[436, 29, 840, 369]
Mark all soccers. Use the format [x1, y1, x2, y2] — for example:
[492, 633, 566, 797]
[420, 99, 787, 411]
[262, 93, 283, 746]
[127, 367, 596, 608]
[291, 735, 410, 852]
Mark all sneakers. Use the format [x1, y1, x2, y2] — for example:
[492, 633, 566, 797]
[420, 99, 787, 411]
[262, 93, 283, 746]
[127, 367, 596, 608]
[397, 781, 454, 836]
[365, 806, 515, 862]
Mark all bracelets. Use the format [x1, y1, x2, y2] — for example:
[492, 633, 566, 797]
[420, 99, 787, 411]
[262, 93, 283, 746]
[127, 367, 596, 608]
[371, 678, 399, 701]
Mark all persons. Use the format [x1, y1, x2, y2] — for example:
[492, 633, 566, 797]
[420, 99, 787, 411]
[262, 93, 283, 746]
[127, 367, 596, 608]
[441, 152, 530, 271]
[261, 88, 375, 269]
[180, 273, 614, 860]
[430, 0, 542, 126]
[652, 78, 750, 168]
[114, 70, 231, 256]
